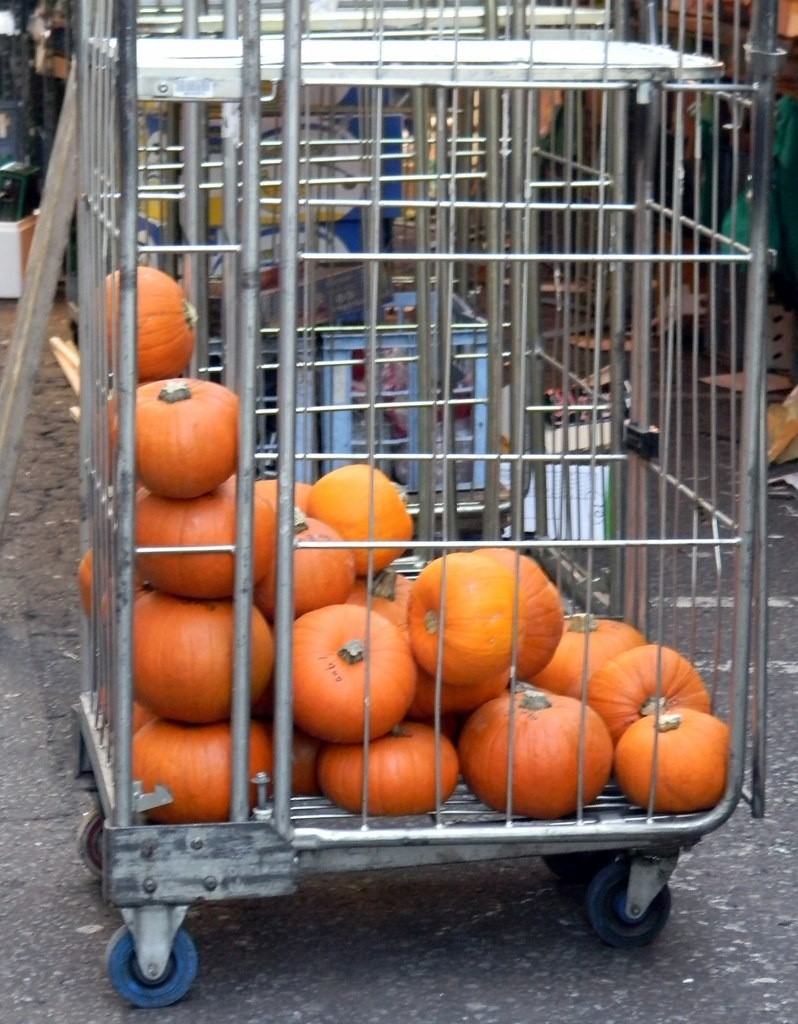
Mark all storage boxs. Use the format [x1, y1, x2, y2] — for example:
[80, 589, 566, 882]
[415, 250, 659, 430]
[0, 211, 39, 299]
[137, 83, 612, 541]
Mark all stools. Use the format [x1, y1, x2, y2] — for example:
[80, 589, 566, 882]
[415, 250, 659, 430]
[0, 162, 40, 220]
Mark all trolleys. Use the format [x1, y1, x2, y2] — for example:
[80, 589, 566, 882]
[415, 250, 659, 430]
[67, 0, 790, 1011]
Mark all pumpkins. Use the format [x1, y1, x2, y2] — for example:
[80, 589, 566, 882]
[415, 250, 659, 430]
[78, 267, 729, 825]
